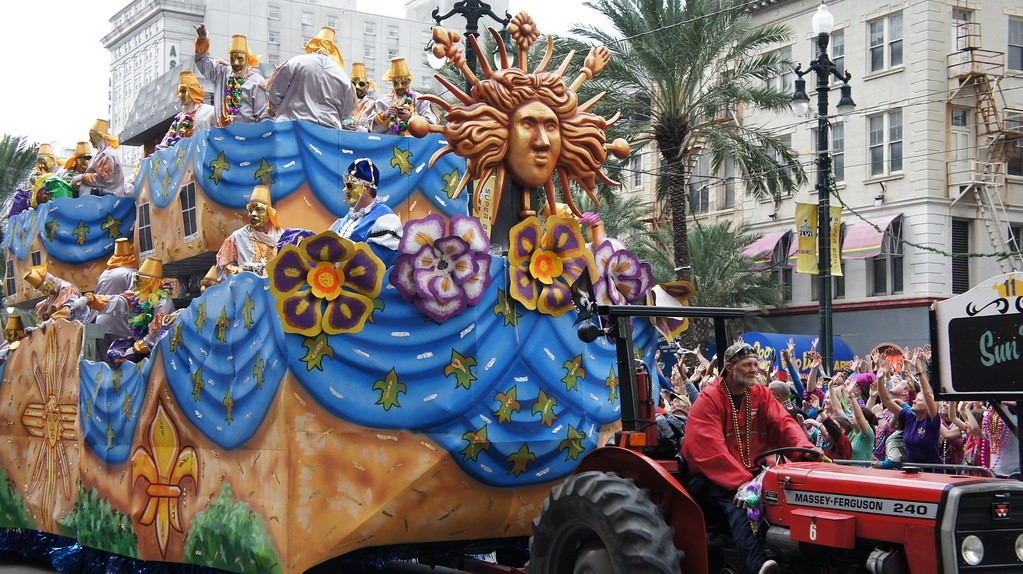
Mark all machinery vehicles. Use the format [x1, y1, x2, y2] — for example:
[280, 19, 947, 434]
[391, 302, 1023, 574]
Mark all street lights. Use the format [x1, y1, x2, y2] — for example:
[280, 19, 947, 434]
[790, 2, 857, 383]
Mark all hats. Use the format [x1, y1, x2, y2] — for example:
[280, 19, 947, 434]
[38, 144, 54, 157]
[388, 57, 410, 78]
[135, 256, 163, 279]
[75, 142, 92, 157]
[348, 157, 379, 190]
[113, 238, 132, 256]
[670, 393, 691, 411]
[227, 35, 248, 55]
[4, 315, 24, 330]
[720, 342, 758, 377]
[250, 185, 273, 208]
[23, 267, 46, 289]
[90, 119, 110, 137]
[178, 71, 199, 88]
[350, 62, 366, 79]
[317, 26, 335, 43]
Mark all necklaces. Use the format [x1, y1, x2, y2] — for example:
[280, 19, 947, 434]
[873, 408, 895, 456]
[816, 431, 823, 450]
[981, 404, 1010, 470]
[168, 113, 193, 147]
[225, 77, 243, 113]
[943, 439, 949, 474]
[724, 381, 752, 468]
[389, 89, 414, 133]
[257, 242, 269, 257]
[782, 400, 788, 404]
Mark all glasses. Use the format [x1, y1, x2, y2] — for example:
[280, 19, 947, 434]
[730, 347, 757, 361]
[344, 183, 356, 188]
[353, 81, 366, 86]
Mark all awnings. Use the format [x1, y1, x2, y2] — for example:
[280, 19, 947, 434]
[738, 213, 903, 270]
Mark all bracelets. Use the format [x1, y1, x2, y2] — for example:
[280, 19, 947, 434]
[817, 409, 823, 413]
[818, 423, 823, 430]
[916, 371, 923, 375]
[878, 379, 884, 383]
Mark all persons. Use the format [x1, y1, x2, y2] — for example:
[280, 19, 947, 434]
[681, 341, 825, 573]
[0, 238, 218, 364]
[655, 335, 1023, 481]
[330, 157, 404, 250]
[217, 186, 284, 279]
[154, 23, 441, 152]
[30, 119, 126, 198]
